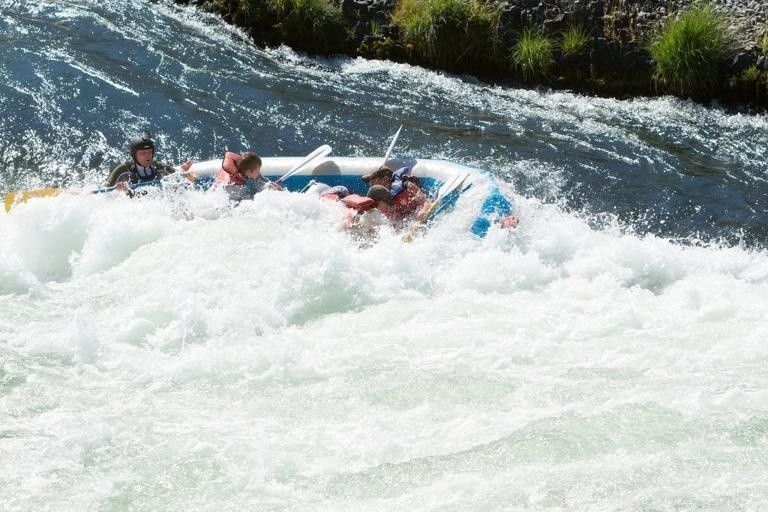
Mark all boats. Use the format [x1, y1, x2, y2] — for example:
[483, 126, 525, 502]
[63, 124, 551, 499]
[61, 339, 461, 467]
[174, 156, 514, 242]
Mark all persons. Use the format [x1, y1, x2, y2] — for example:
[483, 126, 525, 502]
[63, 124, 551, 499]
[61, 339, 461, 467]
[206, 151, 283, 211]
[103, 136, 193, 198]
[319, 165, 429, 249]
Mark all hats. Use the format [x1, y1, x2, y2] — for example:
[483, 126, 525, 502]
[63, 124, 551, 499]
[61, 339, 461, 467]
[361, 165, 393, 182]
[367, 185, 394, 206]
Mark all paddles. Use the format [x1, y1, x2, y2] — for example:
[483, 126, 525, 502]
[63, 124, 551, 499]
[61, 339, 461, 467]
[274, 144, 332, 186]
[382, 125, 402, 166]
[402, 173, 468, 241]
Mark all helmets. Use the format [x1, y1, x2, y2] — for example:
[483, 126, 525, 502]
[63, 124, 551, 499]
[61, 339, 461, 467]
[130, 135, 153, 156]
